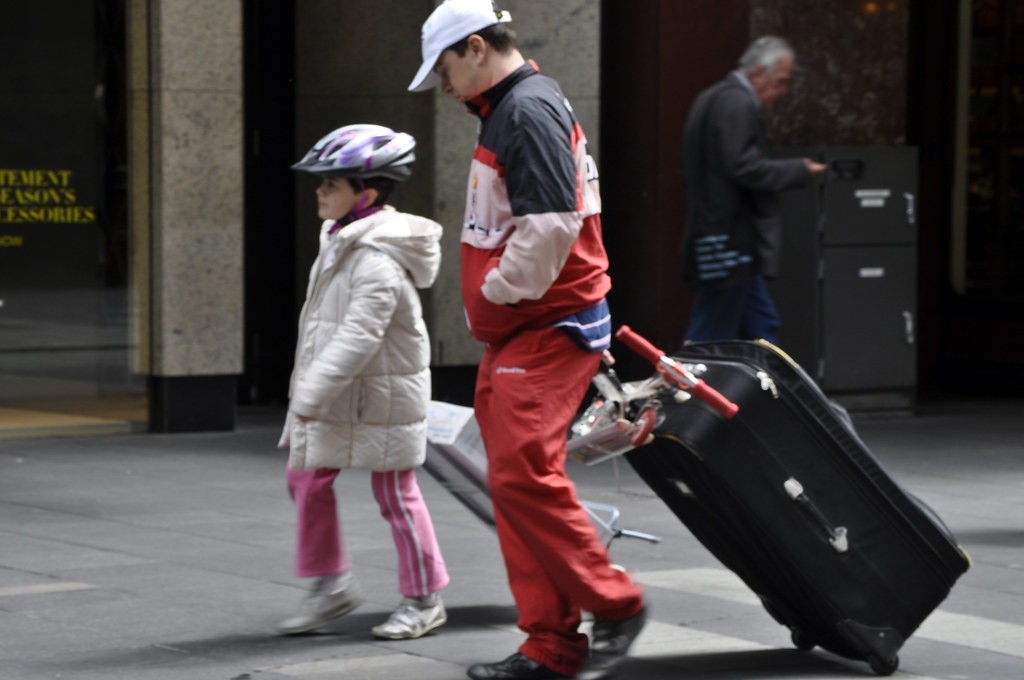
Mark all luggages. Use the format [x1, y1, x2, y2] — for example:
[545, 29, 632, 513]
[593, 325, 970, 676]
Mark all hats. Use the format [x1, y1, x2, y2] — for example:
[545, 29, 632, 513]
[407, 0, 513, 92]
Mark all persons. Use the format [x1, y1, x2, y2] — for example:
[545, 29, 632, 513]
[408, 0, 647, 680]
[676, 35, 827, 349]
[274, 125, 452, 639]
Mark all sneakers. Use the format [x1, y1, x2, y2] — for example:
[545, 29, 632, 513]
[371, 602, 448, 638]
[279, 581, 364, 634]
[584, 606, 648, 676]
[468, 652, 571, 680]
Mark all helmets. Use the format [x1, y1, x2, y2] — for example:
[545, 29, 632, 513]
[290, 124, 416, 182]
